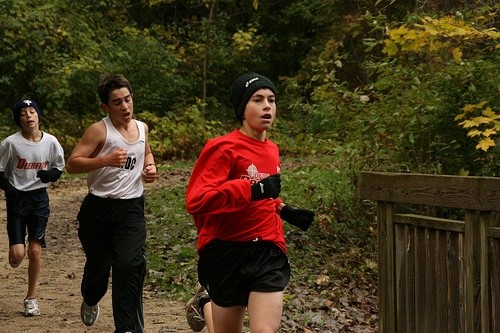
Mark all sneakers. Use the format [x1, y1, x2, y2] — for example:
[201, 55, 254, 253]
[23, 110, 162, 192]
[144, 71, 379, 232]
[81, 299, 99, 326]
[185, 281, 212, 332]
[9, 235, 28, 268]
[23, 297, 41, 317]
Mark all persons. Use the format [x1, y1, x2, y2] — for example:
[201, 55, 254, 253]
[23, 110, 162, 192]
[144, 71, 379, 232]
[65, 75, 156, 333]
[0, 100, 65, 316]
[185, 72, 315, 333]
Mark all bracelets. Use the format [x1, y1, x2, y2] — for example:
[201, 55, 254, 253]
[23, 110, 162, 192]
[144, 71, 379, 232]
[145, 164, 156, 169]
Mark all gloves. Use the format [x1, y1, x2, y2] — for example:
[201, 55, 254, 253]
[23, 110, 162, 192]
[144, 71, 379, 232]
[280, 205, 315, 232]
[0, 171, 10, 190]
[36, 168, 62, 183]
[251, 174, 281, 202]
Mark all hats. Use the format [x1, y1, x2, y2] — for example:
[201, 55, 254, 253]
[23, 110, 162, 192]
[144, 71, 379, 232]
[14, 99, 40, 128]
[230, 72, 276, 120]
